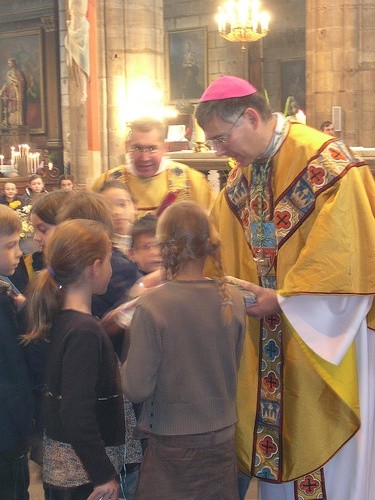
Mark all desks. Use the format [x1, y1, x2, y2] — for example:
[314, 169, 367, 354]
[163, 148, 375, 200]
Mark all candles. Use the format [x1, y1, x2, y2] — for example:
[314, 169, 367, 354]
[31, 154, 34, 174]
[34, 154, 37, 174]
[0, 154, 4, 165]
[37, 153, 40, 168]
[28, 153, 31, 174]
[10, 143, 29, 166]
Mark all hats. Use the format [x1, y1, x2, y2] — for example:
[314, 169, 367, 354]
[200, 74, 257, 101]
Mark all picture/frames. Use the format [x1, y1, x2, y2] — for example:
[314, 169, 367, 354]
[166, 26, 210, 104]
[0, 28, 46, 136]
[279, 58, 306, 115]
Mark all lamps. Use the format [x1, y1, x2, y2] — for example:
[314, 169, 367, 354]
[210, 0, 277, 50]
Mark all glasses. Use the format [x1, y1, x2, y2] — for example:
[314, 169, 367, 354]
[204, 108, 248, 151]
[127, 140, 161, 153]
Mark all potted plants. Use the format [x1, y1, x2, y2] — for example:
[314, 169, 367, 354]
[46, 154, 57, 171]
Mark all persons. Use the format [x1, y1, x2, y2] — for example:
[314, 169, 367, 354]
[283, 97, 306, 126]
[0, 118, 246, 500]
[136, 76, 375, 500]
[321, 121, 336, 137]
